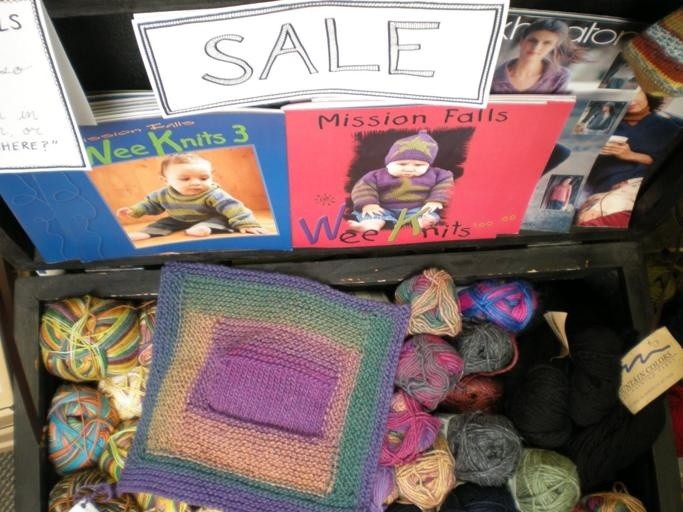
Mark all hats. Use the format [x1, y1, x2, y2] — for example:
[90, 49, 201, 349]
[383, 128, 439, 165]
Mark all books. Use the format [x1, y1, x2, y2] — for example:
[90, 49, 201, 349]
[311, 93, 578, 236]
[0, 87, 294, 265]
[281, 98, 550, 249]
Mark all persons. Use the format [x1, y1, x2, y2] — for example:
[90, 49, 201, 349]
[546, 176, 573, 210]
[583, 101, 616, 134]
[587, 86, 679, 194]
[608, 62, 635, 89]
[346, 129, 455, 236]
[490, 18, 573, 95]
